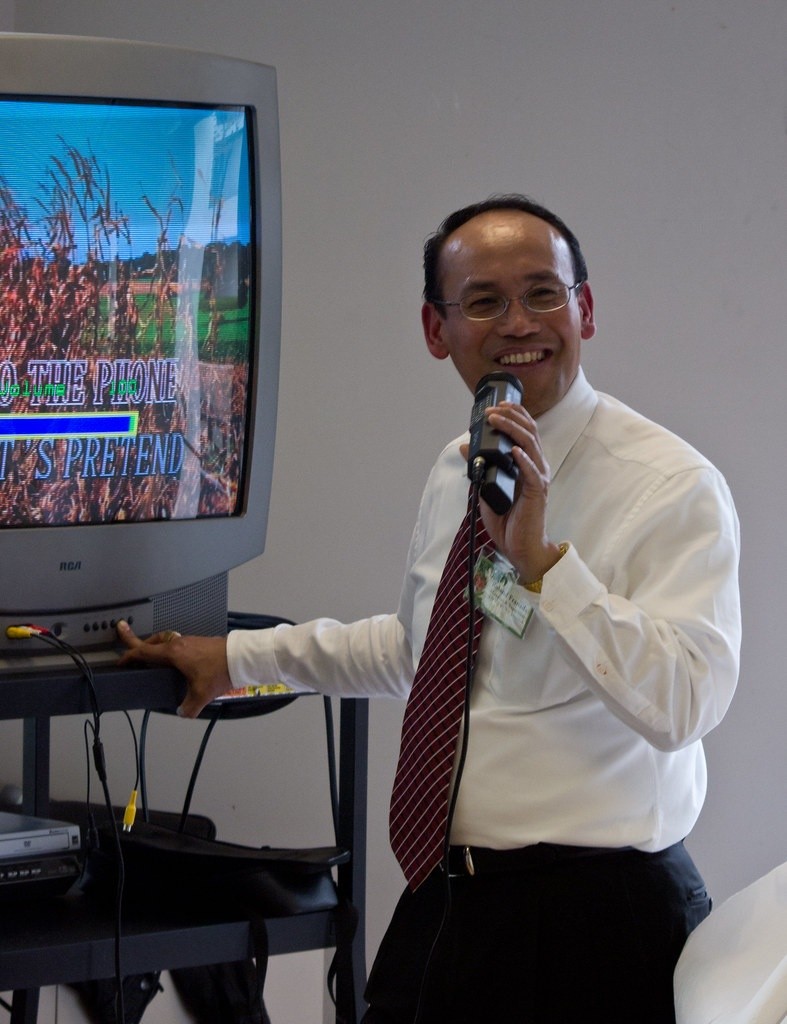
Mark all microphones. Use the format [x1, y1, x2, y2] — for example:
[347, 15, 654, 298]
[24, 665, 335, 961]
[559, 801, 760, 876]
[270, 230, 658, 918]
[468, 371, 524, 517]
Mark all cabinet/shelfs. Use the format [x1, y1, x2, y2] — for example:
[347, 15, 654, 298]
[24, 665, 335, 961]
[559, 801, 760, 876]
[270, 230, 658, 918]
[0, 671, 369, 1024]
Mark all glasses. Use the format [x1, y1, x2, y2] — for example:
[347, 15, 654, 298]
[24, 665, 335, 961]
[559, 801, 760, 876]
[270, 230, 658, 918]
[428, 280, 583, 321]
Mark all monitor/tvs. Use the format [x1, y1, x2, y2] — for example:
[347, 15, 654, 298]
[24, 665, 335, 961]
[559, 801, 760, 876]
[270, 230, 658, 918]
[1, 30, 284, 654]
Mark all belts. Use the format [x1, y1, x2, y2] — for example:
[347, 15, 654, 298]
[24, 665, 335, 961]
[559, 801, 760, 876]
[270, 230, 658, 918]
[430, 844, 635, 881]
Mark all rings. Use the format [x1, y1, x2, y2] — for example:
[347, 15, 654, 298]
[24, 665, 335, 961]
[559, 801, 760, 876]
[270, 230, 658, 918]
[162, 630, 179, 642]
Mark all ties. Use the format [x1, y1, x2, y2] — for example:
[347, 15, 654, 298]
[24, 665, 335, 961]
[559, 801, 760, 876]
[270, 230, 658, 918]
[389, 482, 498, 896]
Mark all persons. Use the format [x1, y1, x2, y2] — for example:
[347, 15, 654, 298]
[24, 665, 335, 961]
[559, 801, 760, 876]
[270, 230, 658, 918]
[116, 195, 742, 1024]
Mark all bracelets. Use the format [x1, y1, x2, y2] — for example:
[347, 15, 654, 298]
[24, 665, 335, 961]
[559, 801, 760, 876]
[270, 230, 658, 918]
[522, 545, 568, 593]
